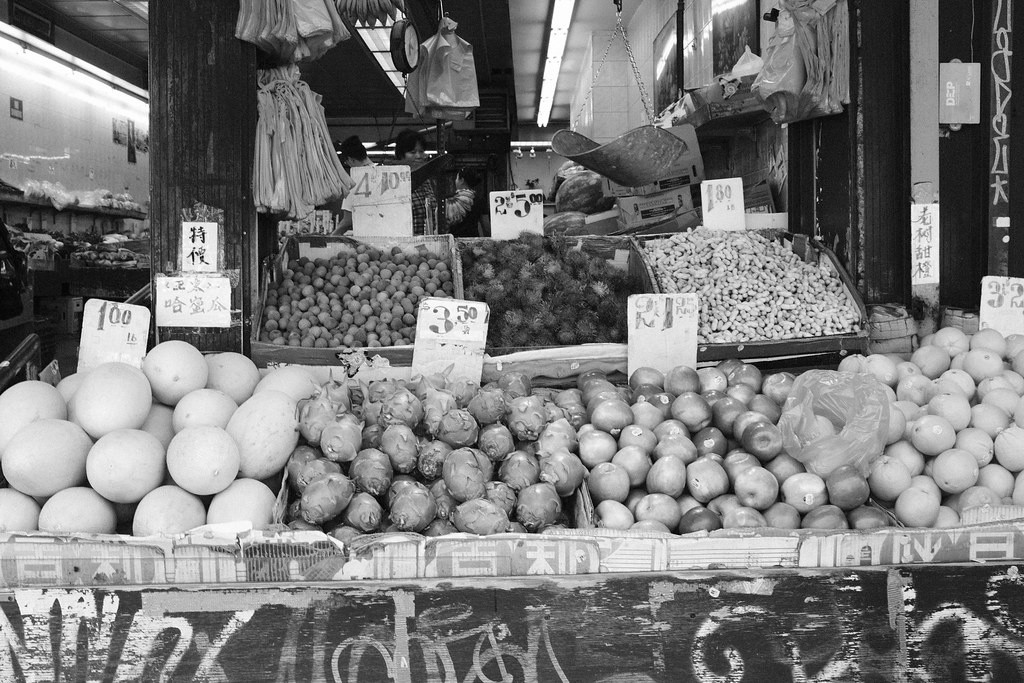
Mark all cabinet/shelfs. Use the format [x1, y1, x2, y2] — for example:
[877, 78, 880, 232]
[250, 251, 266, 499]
[0, 196, 152, 259]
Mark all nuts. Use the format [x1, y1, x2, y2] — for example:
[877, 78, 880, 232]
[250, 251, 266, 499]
[645, 225, 860, 345]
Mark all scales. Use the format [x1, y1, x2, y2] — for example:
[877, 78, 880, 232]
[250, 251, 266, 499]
[370, 19, 454, 196]
[549, 1, 688, 188]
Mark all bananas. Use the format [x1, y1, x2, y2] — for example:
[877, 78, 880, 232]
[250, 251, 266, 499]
[444, 188, 474, 225]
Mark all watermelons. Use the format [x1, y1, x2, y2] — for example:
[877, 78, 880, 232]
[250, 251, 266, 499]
[543, 170, 616, 236]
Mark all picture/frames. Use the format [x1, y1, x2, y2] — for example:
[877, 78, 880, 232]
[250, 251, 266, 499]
[652, 11, 679, 116]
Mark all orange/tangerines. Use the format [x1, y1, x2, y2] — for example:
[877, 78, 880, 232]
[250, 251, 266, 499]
[822, 326, 1023, 530]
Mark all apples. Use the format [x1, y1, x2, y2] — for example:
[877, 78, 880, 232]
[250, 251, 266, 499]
[560, 360, 888, 534]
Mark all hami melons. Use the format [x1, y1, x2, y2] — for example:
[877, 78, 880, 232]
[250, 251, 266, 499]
[0, 338, 318, 534]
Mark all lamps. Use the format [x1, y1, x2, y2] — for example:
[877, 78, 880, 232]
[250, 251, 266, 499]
[537, 0, 576, 128]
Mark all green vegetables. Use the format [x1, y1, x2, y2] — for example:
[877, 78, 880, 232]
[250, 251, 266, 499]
[14, 223, 105, 244]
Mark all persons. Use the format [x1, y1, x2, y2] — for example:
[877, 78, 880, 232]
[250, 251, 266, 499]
[398, 129, 437, 233]
[454, 170, 479, 236]
[329, 136, 386, 236]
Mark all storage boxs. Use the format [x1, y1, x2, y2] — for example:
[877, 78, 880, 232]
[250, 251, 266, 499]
[49, 297, 82, 335]
[583, 124, 705, 235]
[0, 228, 1024, 587]
[70, 265, 151, 299]
[744, 179, 776, 214]
[24, 232, 55, 271]
[656, 73, 758, 121]
